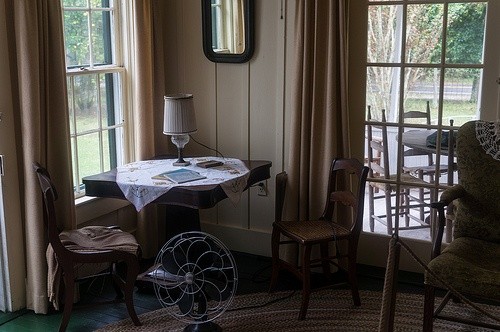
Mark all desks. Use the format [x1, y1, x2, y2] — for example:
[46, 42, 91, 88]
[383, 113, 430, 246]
[396, 128, 457, 217]
[83, 153, 272, 306]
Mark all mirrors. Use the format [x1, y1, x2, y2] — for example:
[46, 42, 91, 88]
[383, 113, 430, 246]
[200, 0, 256, 64]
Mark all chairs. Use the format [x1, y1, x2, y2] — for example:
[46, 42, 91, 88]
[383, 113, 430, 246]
[33, 161, 141, 331]
[265, 101, 500, 332]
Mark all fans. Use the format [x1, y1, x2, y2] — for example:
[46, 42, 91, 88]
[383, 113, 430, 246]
[152, 231, 238, 332]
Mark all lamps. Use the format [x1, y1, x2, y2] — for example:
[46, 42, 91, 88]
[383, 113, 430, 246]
[162, 93, 198, 167]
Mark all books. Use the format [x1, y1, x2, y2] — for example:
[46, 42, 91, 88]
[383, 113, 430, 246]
[151, 169, 207, 184]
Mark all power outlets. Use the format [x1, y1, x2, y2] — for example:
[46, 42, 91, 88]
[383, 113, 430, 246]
[256, 179, 267, 195]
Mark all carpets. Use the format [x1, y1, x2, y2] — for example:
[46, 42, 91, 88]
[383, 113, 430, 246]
[91, 289, 500, 332]
